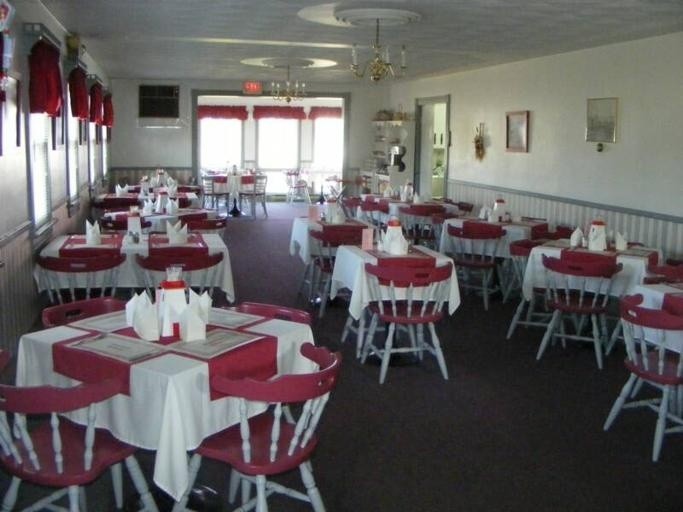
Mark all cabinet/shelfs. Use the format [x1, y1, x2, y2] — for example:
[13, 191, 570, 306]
[360, 119, 415, 194]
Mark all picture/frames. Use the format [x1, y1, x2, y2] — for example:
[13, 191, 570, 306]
[505, 110, 529, 153]
[584, 96, 617, 144]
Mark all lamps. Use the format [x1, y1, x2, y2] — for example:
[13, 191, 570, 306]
[262, 58, 314, 103]
[333, 8, 421, 84]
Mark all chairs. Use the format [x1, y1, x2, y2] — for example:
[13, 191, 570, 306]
[172, 343, 342, 510]
[0, 166, 581, 311]
[222, 300, 312, 326]
[603, 292, 682, 461]
[536, 247, 614, 372]
[40, 294, 127, 329]
[359, 261, 454, 382]
[0, 349, 163, 512]
[605, 261, 682, 358]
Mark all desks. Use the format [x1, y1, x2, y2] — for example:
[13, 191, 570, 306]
[327, 241, 460, 361]
[12, 299, 316, 508]
[506, 237, 665, 344]
[628, 278, 682, 358]
